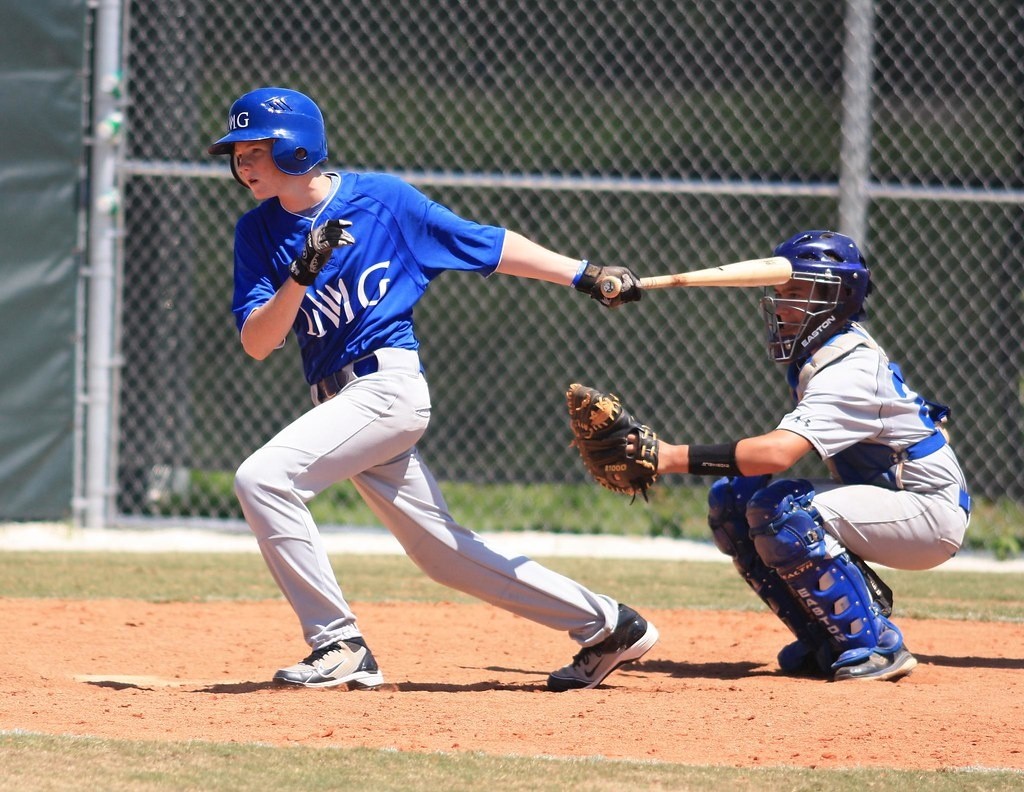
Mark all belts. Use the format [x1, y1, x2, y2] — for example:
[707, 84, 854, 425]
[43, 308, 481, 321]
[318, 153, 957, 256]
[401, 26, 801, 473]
[317, 355, 426, 401]
[959, 489, 971, 520]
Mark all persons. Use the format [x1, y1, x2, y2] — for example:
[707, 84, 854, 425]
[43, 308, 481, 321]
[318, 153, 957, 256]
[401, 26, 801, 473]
[566, 230, 972, 682]
[208, 87, 660, 689]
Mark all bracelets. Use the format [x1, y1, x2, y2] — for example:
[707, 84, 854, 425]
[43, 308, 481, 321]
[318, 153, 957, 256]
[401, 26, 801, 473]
[570, 260, 588, 289]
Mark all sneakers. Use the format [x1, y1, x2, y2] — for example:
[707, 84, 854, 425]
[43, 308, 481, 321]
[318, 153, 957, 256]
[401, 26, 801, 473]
[547, 604, 658, 693]
[835, 641, 917, 681]
[273, 637, 383, 690]
[777, 640, 830, 675]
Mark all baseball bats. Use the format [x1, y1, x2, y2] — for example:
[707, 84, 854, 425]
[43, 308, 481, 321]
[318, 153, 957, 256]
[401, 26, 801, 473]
[600, 255, 793, 299]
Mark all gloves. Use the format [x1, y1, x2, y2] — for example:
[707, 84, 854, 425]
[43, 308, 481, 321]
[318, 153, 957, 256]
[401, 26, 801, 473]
[289, 219, 357, 286]
[570, 259, 641, 307]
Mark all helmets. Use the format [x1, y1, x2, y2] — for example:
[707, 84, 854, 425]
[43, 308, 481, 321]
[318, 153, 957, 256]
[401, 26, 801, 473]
[207, 87, 329, 189]
[762, 230, 871, 364]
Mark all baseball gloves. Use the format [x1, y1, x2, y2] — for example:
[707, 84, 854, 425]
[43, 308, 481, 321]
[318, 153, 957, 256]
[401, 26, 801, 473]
[565, 382, 661, 494]
